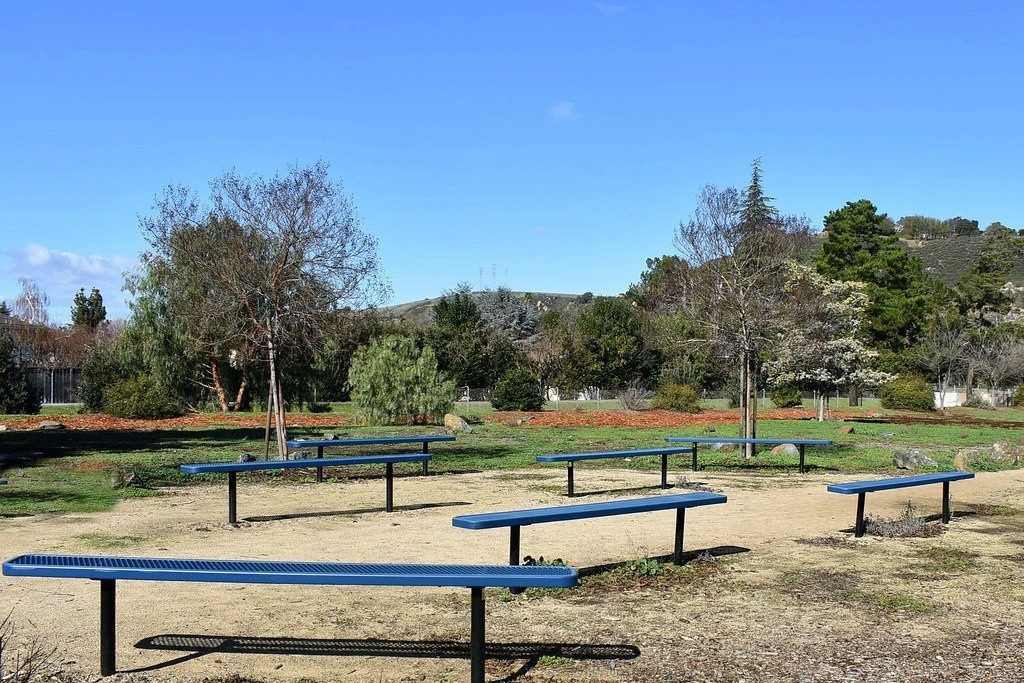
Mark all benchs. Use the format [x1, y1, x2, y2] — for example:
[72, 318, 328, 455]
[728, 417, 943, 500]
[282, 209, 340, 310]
[827, 472, 975, 539]
[285, 436, 456, 483]
[452, 492, 726, 594]
[664, 437, 832, 474]
[536, 448, 693, 497]
[180, 453, 433, 524]
[2, 554, 580, 683]
